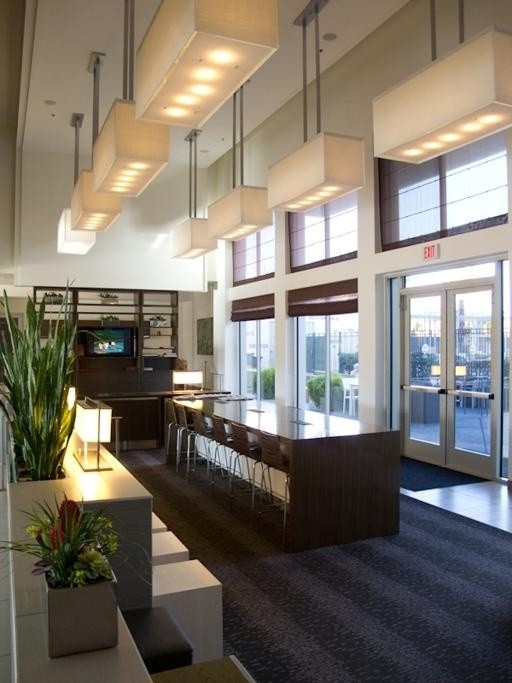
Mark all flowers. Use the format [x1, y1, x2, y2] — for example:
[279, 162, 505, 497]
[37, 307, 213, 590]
[0, 486, 157, 591]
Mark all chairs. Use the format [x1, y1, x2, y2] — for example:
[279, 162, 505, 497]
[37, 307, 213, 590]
[111, 408, 124, 458]
[342, 372, 509, 417]
[163, 399, 293, 528]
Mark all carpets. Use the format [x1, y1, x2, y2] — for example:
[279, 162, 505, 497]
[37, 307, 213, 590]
[117, 446, 512, 683]
[400, 454, 494, 492]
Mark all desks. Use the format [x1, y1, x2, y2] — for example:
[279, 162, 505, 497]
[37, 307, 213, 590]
[163, 393, 401, 554]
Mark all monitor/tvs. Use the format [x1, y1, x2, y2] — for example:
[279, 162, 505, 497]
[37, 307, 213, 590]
[84, 327, 131, 357]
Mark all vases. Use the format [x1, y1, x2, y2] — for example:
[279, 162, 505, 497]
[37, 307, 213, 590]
[38, 541, 118, 659]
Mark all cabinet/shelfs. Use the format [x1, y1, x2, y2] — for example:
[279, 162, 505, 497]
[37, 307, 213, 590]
[33, 285, 179, 359]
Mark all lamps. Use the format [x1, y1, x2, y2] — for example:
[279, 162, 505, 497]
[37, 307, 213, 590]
[206, 79, 273, 242]
[170, 368, 204, 391]
[266, 0, 366, 214]
[72, 395, 114, 472]
[365, 0, 512, 165]
[166, 128, 218, 261]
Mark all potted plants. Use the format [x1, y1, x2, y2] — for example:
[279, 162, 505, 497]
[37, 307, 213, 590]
[0, 286, 80, 482]
